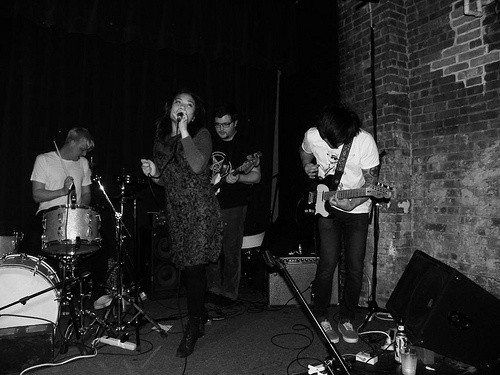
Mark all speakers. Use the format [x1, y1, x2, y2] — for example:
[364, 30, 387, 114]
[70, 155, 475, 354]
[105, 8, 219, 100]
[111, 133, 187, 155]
[267, 256, 339, 306]
[386, 250, 500, 369]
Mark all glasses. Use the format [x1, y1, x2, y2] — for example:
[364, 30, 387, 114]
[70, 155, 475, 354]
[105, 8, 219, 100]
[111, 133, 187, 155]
[215, 121, 233, 128]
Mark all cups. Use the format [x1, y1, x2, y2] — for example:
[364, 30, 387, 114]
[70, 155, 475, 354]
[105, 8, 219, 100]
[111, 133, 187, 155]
[399, 348, 418, 375]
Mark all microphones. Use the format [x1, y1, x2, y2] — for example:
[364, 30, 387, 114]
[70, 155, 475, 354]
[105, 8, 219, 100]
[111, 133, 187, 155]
[70, 184, 77, 204]
[177, 112, 183, 122]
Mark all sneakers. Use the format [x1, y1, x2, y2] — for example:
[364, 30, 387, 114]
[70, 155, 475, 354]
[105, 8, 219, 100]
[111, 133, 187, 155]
[321, 319, 339, 343]
[338, 322, 359, 343]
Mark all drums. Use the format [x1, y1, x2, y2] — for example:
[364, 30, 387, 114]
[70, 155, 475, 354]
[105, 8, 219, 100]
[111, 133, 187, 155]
[0, 251, 63, 336]
[40, 202, 103, 255]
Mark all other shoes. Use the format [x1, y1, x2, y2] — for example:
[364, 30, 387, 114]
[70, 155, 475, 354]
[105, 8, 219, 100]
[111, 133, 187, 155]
[207, 296, 238, 308]
[94, 294, 113, 310]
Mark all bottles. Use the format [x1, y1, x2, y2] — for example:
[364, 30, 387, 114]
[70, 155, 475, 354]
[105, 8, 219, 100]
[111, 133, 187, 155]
[394, 325, 407, 363]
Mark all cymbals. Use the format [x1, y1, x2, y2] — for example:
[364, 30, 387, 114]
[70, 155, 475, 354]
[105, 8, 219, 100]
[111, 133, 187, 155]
[111, 174, 145, 185]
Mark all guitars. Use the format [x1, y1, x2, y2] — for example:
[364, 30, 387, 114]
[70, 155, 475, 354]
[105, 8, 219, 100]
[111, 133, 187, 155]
[210, 152, 262, 192]
[303, 174, 394, 218]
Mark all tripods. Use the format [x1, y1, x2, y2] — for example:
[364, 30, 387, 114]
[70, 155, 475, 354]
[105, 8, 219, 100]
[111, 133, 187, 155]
[59, 170, 168, 355]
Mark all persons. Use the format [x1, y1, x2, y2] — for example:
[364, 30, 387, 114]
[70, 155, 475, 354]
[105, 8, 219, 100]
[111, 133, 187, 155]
[31, 127, 113, 315]
[299, 106, 381, 346]
[140, 89, 260, 355]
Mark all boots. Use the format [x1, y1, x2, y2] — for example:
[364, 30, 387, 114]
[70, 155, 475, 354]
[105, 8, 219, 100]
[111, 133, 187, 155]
[176, 315, 205, 359]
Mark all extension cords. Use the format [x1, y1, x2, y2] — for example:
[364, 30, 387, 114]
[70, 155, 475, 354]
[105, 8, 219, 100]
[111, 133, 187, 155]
[384, 337, 395, 351]
[356, 352, 378, 364]
